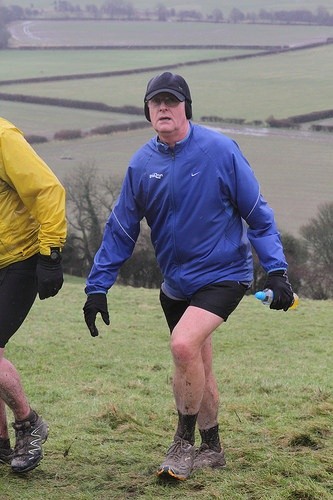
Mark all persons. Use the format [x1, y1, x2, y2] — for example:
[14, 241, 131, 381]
[0, 113, 68, 474]
[83, 71, 294, 481]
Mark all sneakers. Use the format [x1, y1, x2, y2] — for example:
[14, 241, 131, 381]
[0, 410, 49, 473]
[156, 435, 227, 483]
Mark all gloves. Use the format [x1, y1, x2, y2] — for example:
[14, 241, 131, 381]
[263, 274, 295, 312]
[83, 292, 111, 339]
[34, 250, 64, 301]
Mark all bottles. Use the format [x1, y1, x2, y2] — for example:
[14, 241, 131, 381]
[255, 288, 299, 310]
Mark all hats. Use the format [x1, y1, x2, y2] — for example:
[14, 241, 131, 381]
[143, 72, 193, 122]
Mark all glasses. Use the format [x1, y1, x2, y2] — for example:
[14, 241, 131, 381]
[149, 97, 179, 108]
